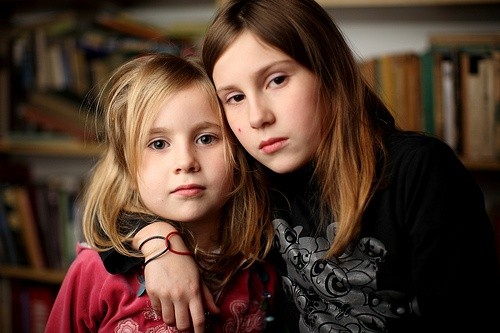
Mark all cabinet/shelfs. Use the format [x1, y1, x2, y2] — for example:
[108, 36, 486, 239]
[0, 0, 500, 332]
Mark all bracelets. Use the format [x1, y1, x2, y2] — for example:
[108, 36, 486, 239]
[138, 230, 195, 267]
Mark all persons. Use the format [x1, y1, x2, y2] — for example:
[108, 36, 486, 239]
[89, 0, 500, 332]
[44, 52, 285, 333]
